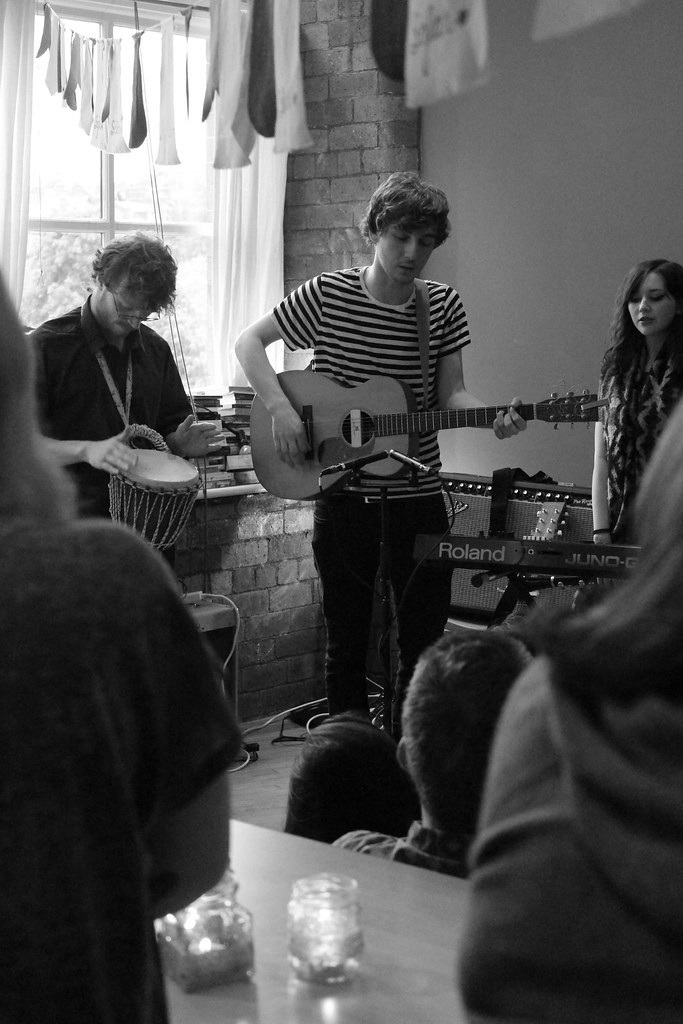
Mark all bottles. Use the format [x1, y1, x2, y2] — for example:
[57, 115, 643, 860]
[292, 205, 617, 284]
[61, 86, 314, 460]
[159, 870, 253, 988]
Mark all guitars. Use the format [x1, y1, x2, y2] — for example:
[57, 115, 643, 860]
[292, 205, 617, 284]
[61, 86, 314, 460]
[249, 369, 611, 501]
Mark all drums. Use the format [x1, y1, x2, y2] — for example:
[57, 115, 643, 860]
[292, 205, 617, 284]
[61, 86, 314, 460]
[106, 447, 203, 553]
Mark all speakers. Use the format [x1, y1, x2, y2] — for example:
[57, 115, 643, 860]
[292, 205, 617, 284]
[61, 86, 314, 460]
[186, 602, 239, 729]
[435, 471, 596, 625]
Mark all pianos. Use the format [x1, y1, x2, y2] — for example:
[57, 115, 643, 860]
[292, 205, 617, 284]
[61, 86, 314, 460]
[413, 528, 642, 635]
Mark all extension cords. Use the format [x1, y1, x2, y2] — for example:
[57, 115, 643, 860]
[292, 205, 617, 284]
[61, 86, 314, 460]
[181, 591, 212, 606]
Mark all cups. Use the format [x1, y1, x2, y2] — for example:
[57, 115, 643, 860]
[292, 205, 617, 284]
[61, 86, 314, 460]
[287, 871, 363, 987]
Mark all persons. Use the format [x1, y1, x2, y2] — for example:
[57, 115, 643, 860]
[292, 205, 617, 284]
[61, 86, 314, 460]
[242, 170, 528, 742]
[31, 236, 225, 569]
[0, 274, 242, 1024]
[285, 632, 533, 881]
[457, 398, 683, 1024]
[590, 256, 682, 544]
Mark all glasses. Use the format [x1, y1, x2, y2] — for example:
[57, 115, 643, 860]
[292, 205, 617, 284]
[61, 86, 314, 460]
[108, 289, 162, 322]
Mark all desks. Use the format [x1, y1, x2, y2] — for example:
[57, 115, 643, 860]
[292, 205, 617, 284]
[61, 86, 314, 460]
[153, 816, 495, 1024]
